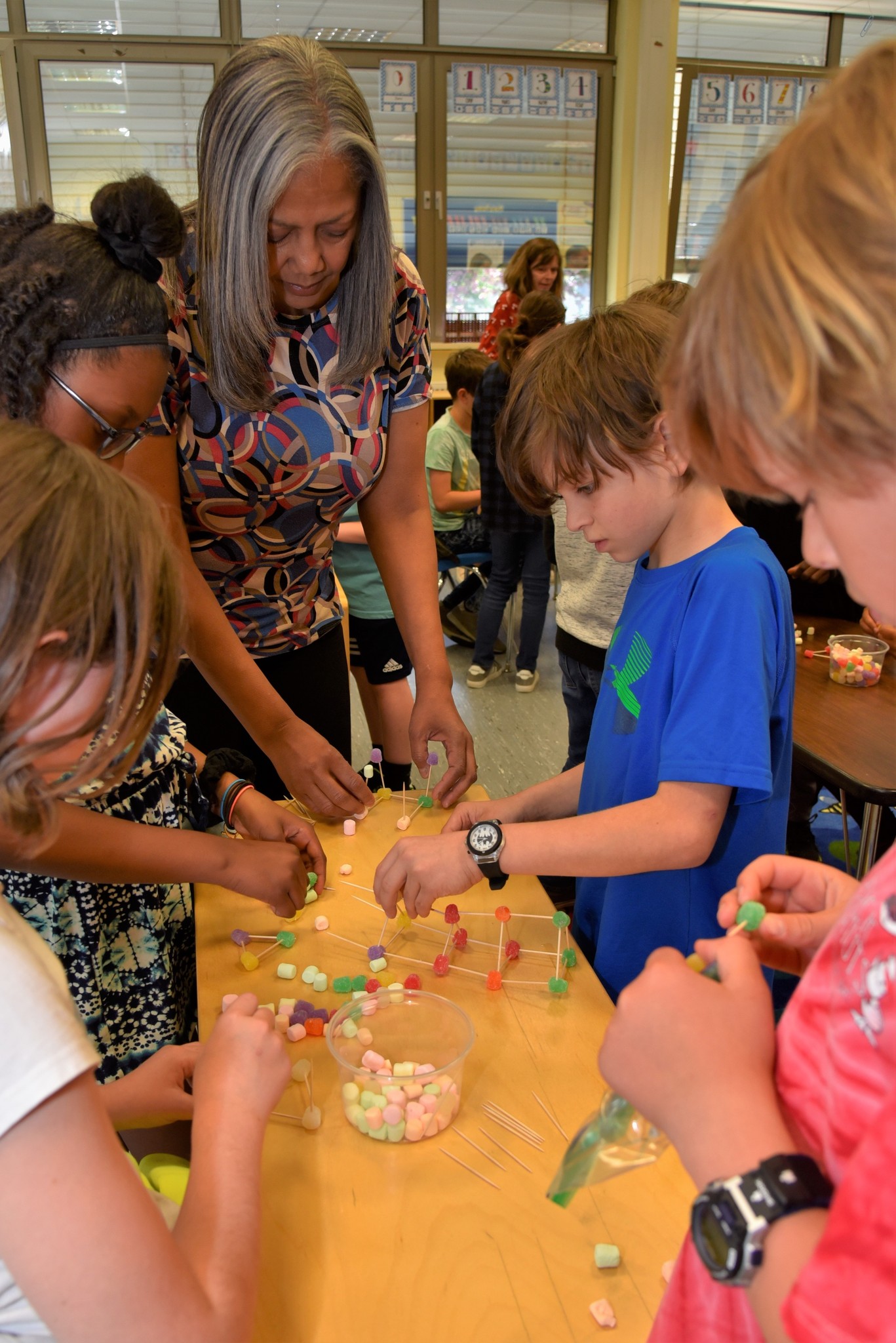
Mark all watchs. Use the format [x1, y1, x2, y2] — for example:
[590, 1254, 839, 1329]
[463, 818, 509, 891]
[690, 1152, 836, 1288]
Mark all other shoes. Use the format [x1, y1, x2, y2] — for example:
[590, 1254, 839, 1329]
[439, 600, 476, 648]
[466, 660, 505, 688]
[356, 761, 417, 793]
[446, 601, 507, 653]
[515, 670, 539, 692]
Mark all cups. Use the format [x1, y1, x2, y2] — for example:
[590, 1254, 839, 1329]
[826, 633, 890, 687]
[325, 988, 473, 1144]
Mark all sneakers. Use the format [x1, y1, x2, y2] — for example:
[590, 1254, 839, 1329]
[786, 776, 821, 864]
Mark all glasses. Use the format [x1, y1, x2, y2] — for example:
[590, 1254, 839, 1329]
[45, 367, 152, 459]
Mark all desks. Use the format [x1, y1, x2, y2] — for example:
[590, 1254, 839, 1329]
[792, 617, 896, 879]
[196, 786, 697, 1343]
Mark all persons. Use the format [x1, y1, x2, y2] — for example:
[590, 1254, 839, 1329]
[124, 33, 478, 799]
[0, 171, 414, 1166]
[598, 40, 896, 1343]
[0, 418, 297, 1343]
[373, 297, 798, 1022]
[425, 232, 896, 918]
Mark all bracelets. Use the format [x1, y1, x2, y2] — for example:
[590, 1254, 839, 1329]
[193, 748, 255, 829]
[219, 778, 255, 840]
